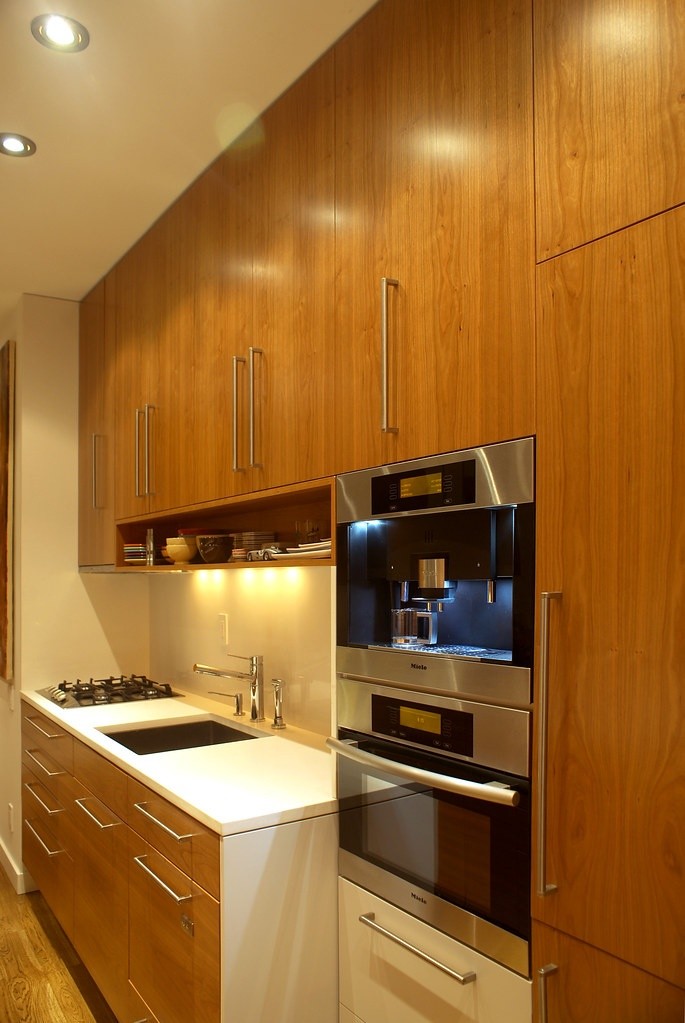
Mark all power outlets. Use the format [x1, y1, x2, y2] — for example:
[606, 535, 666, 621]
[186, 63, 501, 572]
[218, 613, 229, 645]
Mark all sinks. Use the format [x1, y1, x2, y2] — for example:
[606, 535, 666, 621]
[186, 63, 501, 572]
[96, 712, 273, 756]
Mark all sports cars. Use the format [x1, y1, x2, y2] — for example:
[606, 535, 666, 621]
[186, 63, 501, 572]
[247, 546, 284, 562]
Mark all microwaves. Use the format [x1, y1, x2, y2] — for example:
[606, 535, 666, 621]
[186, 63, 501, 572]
[334, 436, 539, 709]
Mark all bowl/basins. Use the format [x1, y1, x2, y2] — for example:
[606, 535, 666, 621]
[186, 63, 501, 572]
[161, 534, 235, 565]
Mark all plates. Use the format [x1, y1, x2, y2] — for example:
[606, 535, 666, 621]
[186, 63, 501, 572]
[123, 544, 165, 565]
[271, 537, 332, 560]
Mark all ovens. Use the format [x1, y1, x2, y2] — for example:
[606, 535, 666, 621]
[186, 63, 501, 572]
[325, 643, 535, 1023]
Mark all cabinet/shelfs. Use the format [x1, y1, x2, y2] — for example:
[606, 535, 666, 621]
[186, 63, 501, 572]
[0, 677, 39, 895]
[336, 875, 532, 1023]
[126, 776, 339, 1023]
[530, 918, 685, 1023]
[528, 203, 684, 992]
[77, 276, 174, 574]
[103, 179, 193, 528]
[73, 737, 128, 1018]
[531, 0, 685, 264]
[190, 43, 335, 516]
[323, 0, 535, 475]
[20, 699, 75, 946]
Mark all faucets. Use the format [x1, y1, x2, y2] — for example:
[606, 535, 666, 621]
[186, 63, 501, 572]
[193, 654, 264, 720]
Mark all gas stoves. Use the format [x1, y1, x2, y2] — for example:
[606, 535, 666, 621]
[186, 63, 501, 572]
[34, 675, 185, 709]
